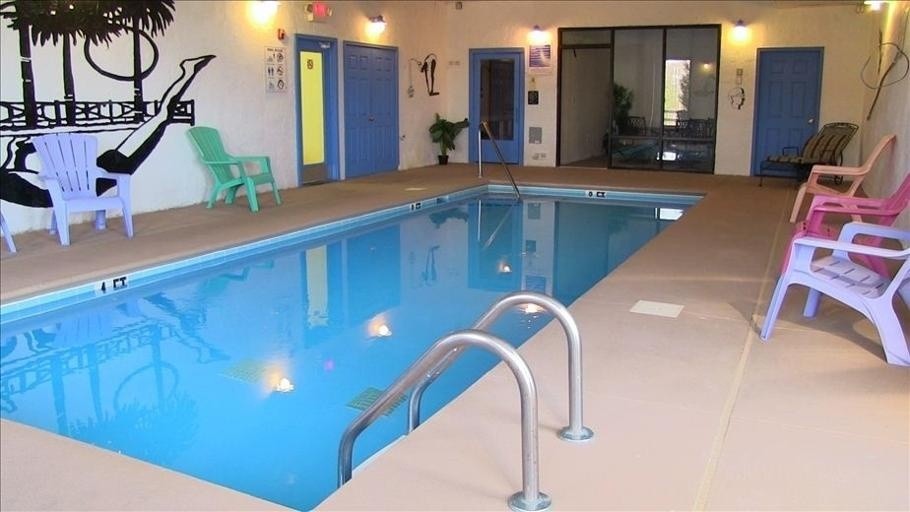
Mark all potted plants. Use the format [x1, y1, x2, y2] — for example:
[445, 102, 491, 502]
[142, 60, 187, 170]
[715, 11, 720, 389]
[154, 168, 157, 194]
[428, 113, 469, 165]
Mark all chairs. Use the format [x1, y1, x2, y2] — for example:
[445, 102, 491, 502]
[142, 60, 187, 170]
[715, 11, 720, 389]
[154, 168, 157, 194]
[31, 133, 134, 247]
[760, 122, 910, 368]
[616, 110, 716, 170]
[185, 126, 282, 213]
